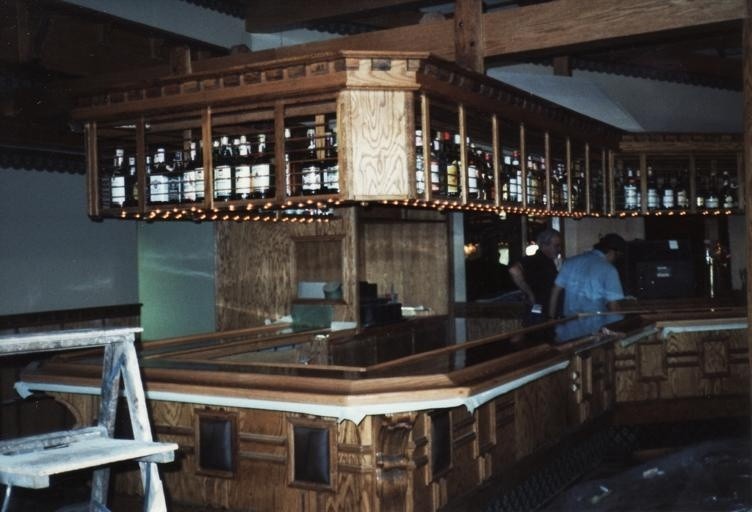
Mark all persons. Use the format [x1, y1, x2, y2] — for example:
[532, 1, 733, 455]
[465, 239, 519, 302]
[545, 233, 627, 343]
[502, 228, 564, 341]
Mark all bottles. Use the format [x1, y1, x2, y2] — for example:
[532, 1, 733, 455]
[623, 164, 736, 210]
[282, 127, 340, 196]
[213, 132, 274, 201]
[108, 138, 204, 207]
[414, 128, 587, 210]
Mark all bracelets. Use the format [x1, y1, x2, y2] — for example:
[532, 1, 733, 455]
[547, 317, 557, 319]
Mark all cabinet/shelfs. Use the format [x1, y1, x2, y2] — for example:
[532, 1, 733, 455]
[82, 52, 336, 221]
[620, 131, 751, 220]
[336, 52, 621, 220]
